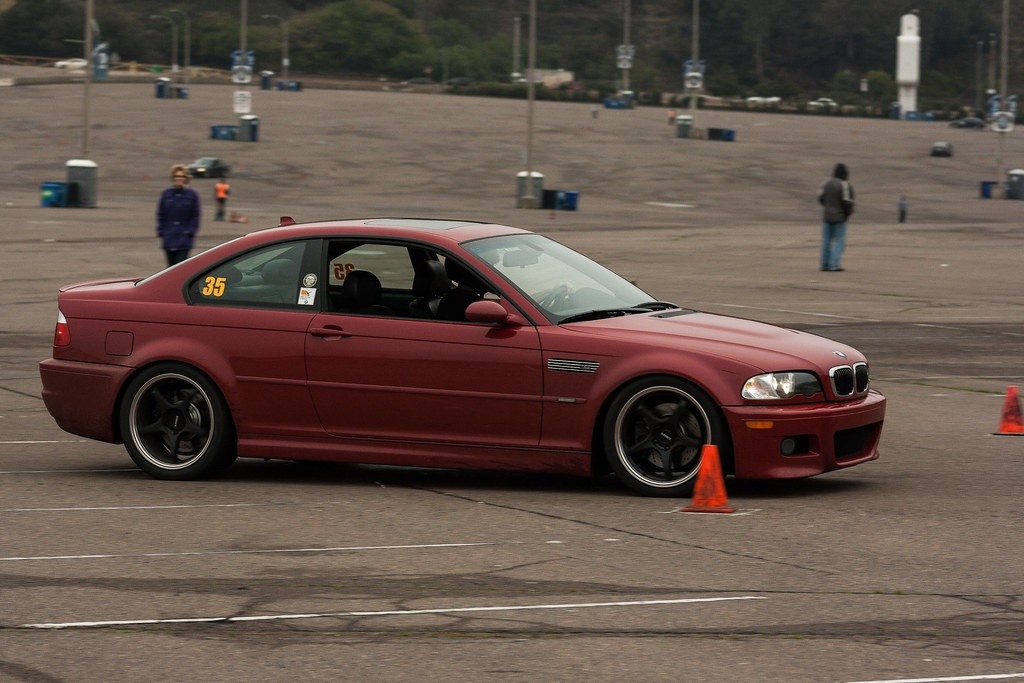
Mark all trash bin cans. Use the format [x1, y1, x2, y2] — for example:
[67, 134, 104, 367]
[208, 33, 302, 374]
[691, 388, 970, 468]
[65, 159, 99, 208]
[259, 71, 275, 88]
[1007, 169, 1024, 198]
[238, 116, 260, 143]
[155, 76, 171, 97]
[676, 115, 693, 138]
[516, 170, 545, 209]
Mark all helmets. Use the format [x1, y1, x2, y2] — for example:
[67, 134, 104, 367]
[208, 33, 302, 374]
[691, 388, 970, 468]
[445, 241, 500, 285]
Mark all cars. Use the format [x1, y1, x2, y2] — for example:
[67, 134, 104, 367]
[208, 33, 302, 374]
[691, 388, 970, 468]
[188, 156, 231, 178]
[38, 215, 888, 501]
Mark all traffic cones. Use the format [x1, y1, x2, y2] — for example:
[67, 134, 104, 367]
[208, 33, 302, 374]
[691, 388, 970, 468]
[991, 384, 1024, 436]
[680, 444, 736, 514]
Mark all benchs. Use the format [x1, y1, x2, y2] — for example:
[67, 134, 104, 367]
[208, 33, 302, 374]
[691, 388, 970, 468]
[208, 258, 301, 306]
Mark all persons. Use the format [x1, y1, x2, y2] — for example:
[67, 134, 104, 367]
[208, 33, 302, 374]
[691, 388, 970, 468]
[817, 163, 856, 272]
[436, 249, 578, 324]
[214, 177, 231, 220]
[155, 164, 200, 268]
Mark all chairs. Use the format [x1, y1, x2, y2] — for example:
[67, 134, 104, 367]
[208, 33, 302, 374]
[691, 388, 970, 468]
[409, 262, 457, 317]
[334, 271, 386, 314]
